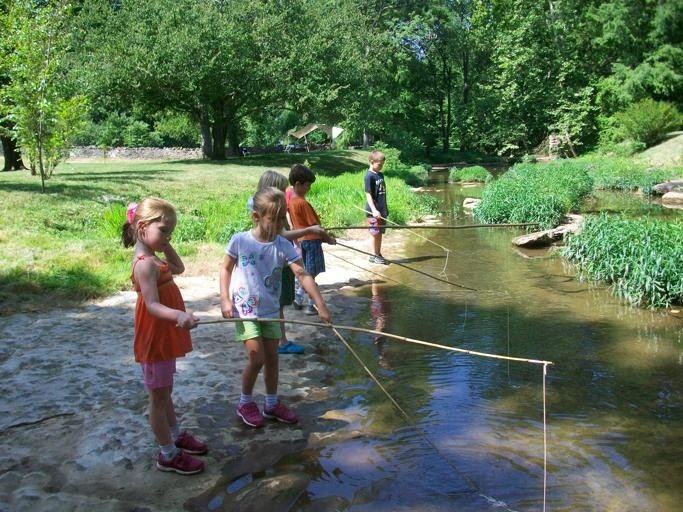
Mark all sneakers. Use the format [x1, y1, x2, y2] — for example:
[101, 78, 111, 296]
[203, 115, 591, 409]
[156, 448, 204, 475]
[262, 400, 298, 424]
[293, 294, 302, 310]
[236, 401, 264, 428]
[277, 341, 304, 354]
[369, 255, 385, 264]
[174, 431, 207, 454]
[305, 306, 317, 314]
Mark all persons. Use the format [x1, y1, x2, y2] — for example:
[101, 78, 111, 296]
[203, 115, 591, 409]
[364, 150, 389, 265]
[288, 166, 336, 315]
[119, 195, 208, 475]
[255, 169, 328, 354]
[219, 186, 332, 428]
[285, 163, 300, 251]
